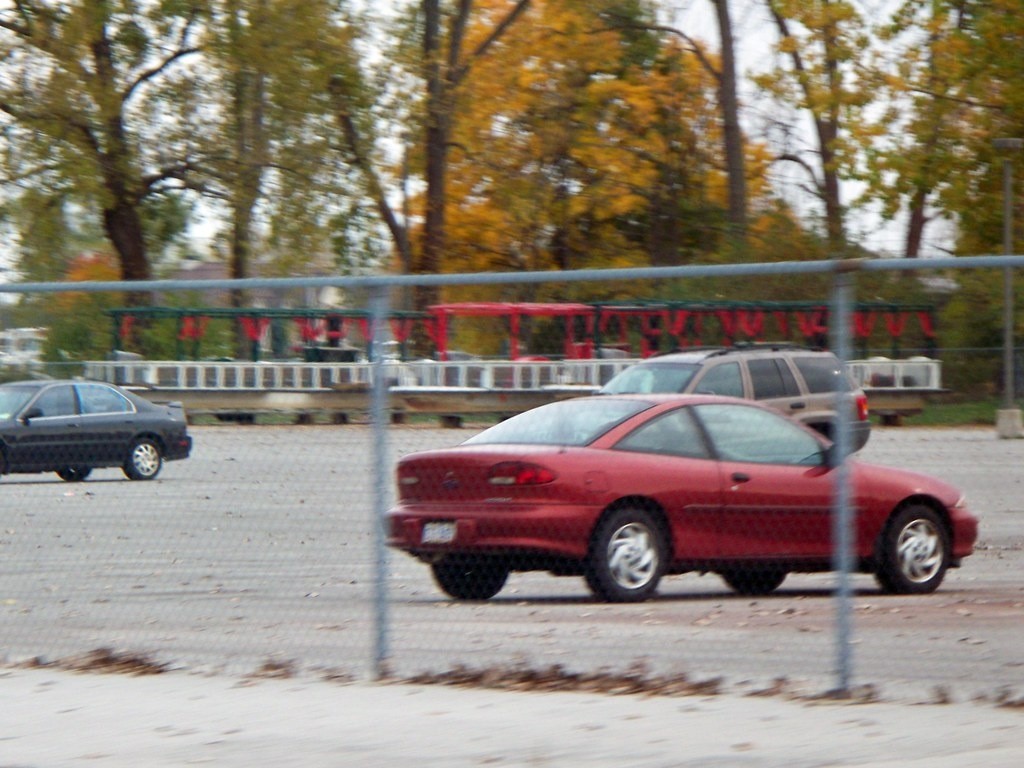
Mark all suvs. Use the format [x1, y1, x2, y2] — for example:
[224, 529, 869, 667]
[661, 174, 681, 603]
[599, 342, 873, 460]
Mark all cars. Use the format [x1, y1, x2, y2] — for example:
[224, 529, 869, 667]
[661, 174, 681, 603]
[386, 393, 978, 604]
[0, 380, 194, 481]
